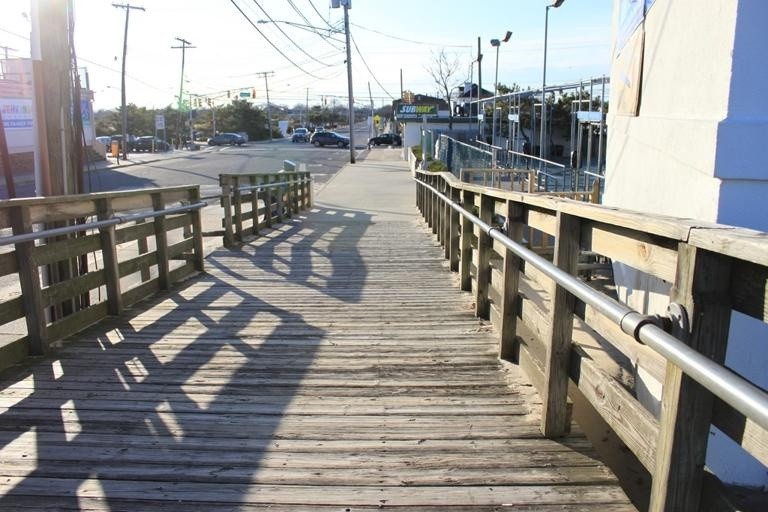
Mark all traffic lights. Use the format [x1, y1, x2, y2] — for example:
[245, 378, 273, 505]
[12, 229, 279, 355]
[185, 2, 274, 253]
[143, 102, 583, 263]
[197, 98, 202, 108]
[207, 97, 212, 107]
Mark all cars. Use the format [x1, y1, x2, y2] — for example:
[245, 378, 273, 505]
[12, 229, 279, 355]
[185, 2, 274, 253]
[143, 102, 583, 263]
[206, 132, 246, 146]
[369, 133, 401, 146]
[96, 134, 170, 152]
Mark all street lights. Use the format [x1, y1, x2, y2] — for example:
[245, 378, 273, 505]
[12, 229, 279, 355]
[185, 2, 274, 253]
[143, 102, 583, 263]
[535, 0, 564, 183]
[468, 54, 483, 127]
[489, 31, 513, 146]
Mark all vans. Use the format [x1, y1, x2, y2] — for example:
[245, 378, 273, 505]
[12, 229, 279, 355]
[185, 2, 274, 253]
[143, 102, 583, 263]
[291, 127, 311, 142]
[309, 131, 350, 147]
[314, 126, 326, 135]
